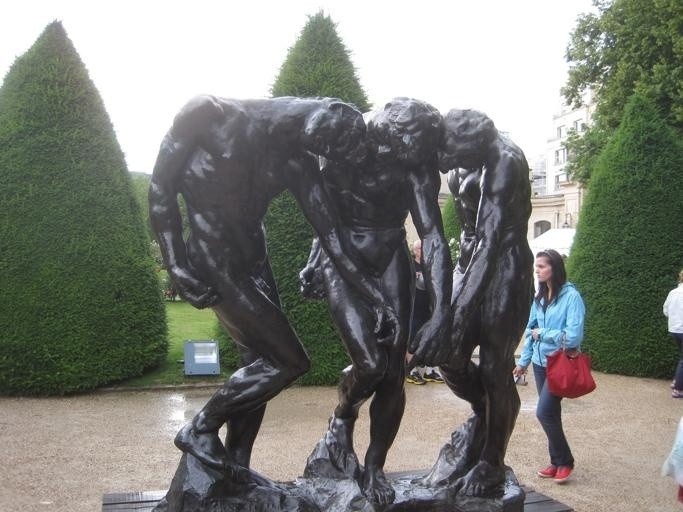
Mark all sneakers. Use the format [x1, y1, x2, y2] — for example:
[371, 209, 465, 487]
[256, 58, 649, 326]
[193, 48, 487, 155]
[406, 370, 426, 385]
[537, 464, 557, 478]
[423, 369, 445, 383]
[553, 465, 574, 482]
[670, 380, 683, 398]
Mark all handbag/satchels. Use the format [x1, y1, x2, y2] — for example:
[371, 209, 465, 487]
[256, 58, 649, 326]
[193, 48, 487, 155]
[544, 332, 597, 399]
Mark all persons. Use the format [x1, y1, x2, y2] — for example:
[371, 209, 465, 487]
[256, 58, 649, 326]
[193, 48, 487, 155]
[511, 248, 587, 485]
[146, 93, 368, 485]
[293, 97, 456, 509]
[404, 346, 443, 387]
[433, 106, 536, 498]
[661, 270, 683, 401]
